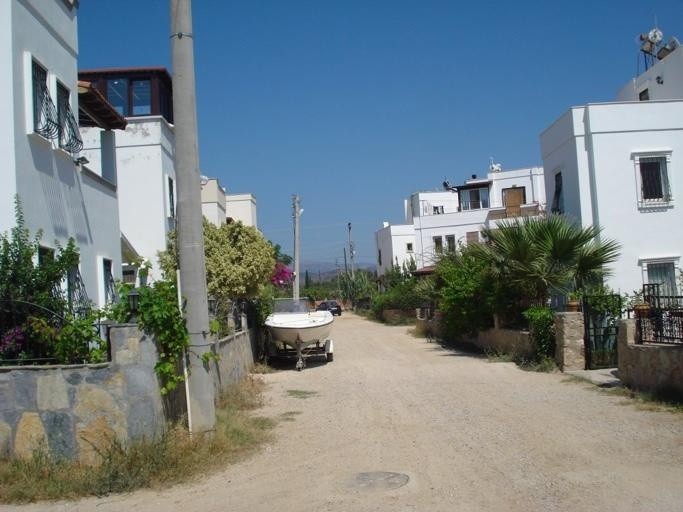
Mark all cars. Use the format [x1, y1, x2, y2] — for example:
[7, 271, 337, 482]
[313, 300, 341, 315]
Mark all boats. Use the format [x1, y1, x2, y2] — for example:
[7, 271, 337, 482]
[263, 292, 334, 372]
